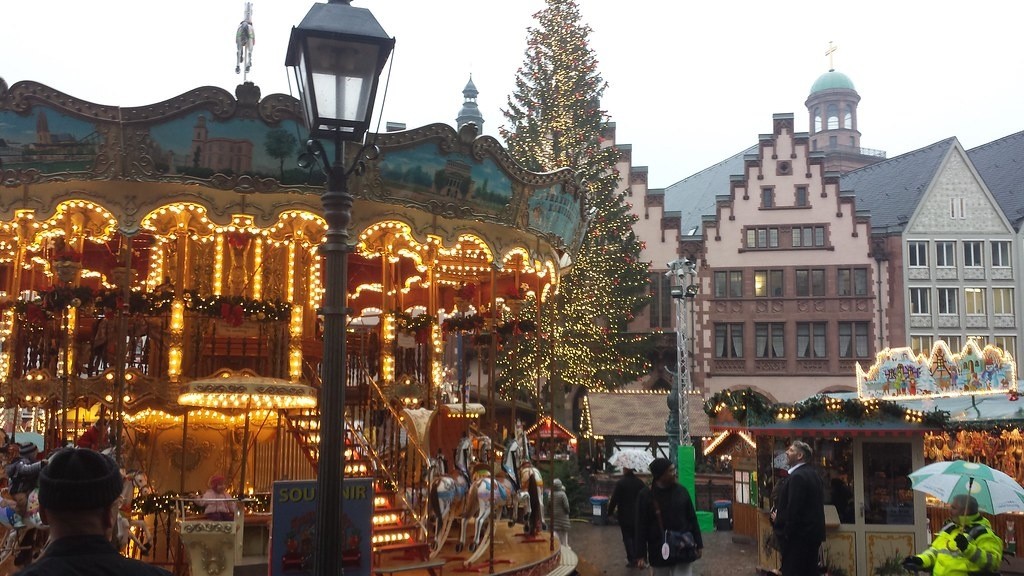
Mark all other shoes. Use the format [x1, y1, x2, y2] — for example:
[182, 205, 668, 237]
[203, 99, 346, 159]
[625, 561, 649, 568]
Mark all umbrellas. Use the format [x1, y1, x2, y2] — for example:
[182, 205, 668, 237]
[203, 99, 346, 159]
[607, 447, 656, 475]
[907, 459, 1024, 536]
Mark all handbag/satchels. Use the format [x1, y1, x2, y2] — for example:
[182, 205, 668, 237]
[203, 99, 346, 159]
[662, 530, 699, 563]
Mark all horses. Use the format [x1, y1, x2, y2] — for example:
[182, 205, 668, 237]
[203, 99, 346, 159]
[417, 430, 548, 568]
[0, 428, 154, 568]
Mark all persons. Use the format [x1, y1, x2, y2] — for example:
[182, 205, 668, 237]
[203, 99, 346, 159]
[902, 495, 1003, 575]
[11, 447, 176, 576]
[187, 472, 237, 521]
[549, 479, 571, 547]
[6, 442, 49, 516]
[768, 440, 827, 576]
[606, 468, 646, 568]
[637, 458, 704, 576]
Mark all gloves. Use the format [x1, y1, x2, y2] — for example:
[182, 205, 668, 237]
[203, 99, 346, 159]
[955, 534, 969, 552]
[900, 555, 923, 573]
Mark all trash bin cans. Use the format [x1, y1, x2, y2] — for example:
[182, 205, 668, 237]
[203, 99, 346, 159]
[590, 495, 609, 525]
[715, 500, 731, 531]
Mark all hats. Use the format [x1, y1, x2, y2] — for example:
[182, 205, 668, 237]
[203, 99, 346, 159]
[649, 459, 674, 478]
[39, 446, 122, 510]
